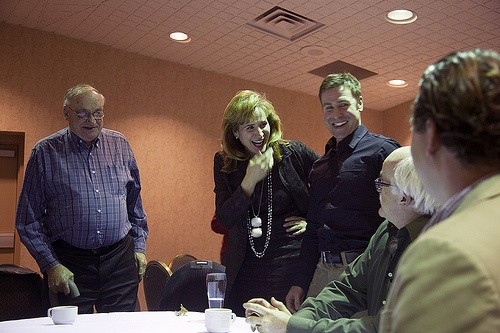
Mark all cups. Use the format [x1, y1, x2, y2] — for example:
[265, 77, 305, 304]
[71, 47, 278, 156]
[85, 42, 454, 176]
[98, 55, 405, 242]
[206, 272, 227, 309]
[47, 306, 78, 326]
[205, 308, 236, 333]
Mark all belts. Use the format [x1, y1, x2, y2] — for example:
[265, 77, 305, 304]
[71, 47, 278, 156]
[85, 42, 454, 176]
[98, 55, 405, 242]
[318, 249, 365, 264]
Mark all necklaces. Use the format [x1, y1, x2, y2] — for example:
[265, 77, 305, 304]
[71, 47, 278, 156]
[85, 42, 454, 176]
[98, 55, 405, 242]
[250, 175, 265, 237]
[246, 164, 273, 259]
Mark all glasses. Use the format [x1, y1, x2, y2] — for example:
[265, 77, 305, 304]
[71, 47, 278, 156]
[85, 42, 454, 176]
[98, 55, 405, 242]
[375, 177, 391, 192]
[67, 106, 104, 120]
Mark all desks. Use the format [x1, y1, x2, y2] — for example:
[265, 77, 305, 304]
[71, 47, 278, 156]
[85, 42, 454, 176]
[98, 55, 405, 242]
[0, 311, 259, 333]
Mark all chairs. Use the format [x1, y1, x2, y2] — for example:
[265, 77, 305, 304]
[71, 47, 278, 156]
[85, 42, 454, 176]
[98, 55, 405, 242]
[143, 252, 226, 313]
[0, 263, 50, 322]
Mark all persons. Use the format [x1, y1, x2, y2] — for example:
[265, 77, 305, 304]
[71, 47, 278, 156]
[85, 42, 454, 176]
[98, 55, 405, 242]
[15, 83, 148, 314]
[381, 50, 500, 332]
[168, 255, 198, 273]
[243, 145, 441, 333]
[285, 73, 401, 315]
[211, 91, 322, 317]
